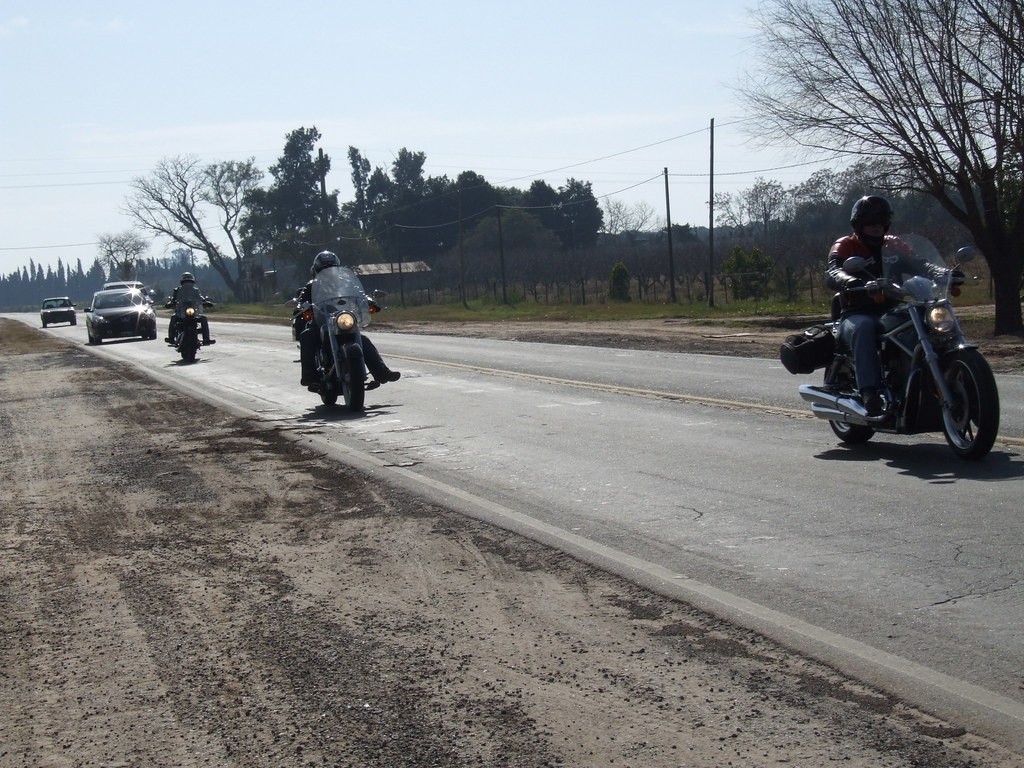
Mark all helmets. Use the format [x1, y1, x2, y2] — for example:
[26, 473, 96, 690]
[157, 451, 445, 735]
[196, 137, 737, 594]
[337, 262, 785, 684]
[180, 272, 196, 286]
[850, 196, 894, 232]
[314, 251, 340, 271]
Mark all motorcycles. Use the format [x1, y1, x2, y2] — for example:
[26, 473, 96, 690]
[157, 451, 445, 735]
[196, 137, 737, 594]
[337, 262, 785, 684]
[291, 265, 390, 414]
[167, 282, 213, 363]
[798, 236, 1000, 464]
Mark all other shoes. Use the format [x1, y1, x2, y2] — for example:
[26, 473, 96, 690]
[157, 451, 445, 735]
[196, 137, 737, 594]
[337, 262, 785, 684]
[301, 375, 321, 386]
[164, 338, 175, 344]
[203, 339, 216, 346]
[862, 394, 883, 416]
[376, 370, 401, 384]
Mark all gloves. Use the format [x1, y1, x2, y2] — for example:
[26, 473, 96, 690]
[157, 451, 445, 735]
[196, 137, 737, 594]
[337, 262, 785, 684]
[208, 301, 213, 307]
[952, 270, 965, 286]
[165, 302, 172, 309]
[847, 278, 865, 296]
[371, 302, 382, 313]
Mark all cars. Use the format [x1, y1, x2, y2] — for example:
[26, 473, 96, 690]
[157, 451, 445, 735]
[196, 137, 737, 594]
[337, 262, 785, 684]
[83, 288, 157, 345]
[101, 281, 156, 315]
[40, 297, 77, 328]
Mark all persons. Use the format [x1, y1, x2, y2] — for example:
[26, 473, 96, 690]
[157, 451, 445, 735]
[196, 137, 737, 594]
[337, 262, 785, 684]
[60, 300, 68, 306]
[298, 252, 400, 386]
[165, 272, 216, 345]
[827, 196, 964, 416]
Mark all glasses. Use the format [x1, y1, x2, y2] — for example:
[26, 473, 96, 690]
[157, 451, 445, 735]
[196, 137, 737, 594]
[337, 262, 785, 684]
[866, 218, 891, 227]
